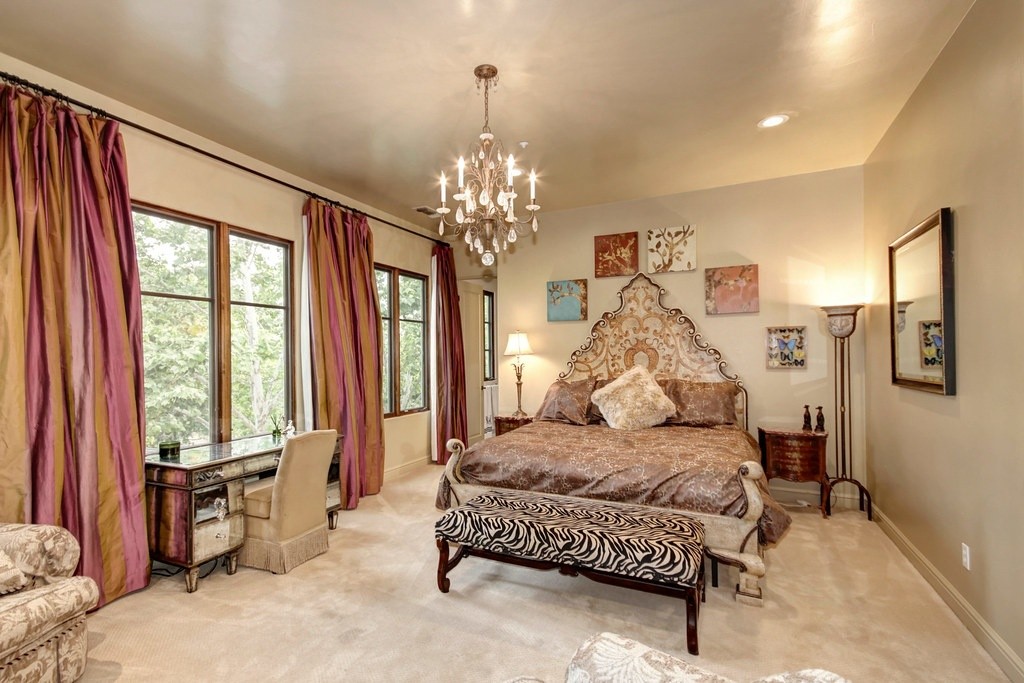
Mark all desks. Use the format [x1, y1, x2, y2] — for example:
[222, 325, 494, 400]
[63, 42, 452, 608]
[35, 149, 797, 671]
[145, 429, 344, 593]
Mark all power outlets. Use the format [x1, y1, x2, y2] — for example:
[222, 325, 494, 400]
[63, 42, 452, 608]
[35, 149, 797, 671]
[963, 543, 969, 571]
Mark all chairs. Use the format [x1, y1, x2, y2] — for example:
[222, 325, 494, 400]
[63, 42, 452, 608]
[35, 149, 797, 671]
[235, 429, 338, 574]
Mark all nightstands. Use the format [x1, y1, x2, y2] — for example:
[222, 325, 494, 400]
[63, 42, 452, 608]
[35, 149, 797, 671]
[494, 416, 533, 437]
[756, 426, 829, 519]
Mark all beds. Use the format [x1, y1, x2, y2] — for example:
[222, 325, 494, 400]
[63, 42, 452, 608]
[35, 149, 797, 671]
[435, 271, 792, 608]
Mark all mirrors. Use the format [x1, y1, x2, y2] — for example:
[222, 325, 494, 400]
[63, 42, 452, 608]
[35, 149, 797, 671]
[888, 207, 957, 396]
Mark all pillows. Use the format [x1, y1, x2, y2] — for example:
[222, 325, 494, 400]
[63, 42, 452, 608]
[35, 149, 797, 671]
[661, 379, 741, 427]
[591, 364, 676, 431]
[532, 374, 598, 426]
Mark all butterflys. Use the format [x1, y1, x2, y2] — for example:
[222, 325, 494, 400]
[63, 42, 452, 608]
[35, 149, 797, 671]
[922, 322, 943, 366]
[768, 327, 804, 368]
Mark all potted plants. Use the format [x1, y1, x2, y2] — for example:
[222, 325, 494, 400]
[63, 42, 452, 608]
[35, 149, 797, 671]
[270, 413, 284, 436]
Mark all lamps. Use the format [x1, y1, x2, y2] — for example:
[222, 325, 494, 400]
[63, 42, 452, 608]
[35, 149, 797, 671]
[820, 277, 872, 520]
[896, 301, 914, 334]
[502, 330, 534, 417]
[435, 64, 541, 267]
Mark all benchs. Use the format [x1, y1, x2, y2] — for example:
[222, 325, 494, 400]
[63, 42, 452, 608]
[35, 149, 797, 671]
[435, 491, 707, 656]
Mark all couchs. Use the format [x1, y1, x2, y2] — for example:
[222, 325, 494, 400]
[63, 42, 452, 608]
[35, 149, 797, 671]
[0, 523, 100, 683]
